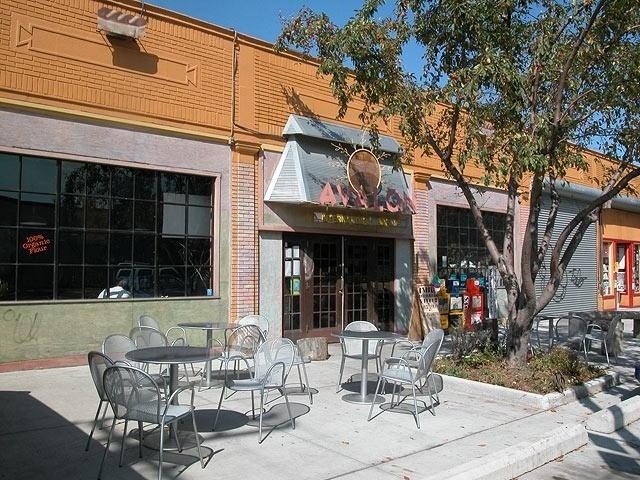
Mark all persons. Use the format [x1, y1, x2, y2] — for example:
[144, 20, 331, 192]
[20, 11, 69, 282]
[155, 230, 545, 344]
[97, 277, 133, 299]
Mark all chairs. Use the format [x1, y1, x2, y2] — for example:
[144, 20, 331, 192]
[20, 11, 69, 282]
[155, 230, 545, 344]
[85, 315, 313, 480]
[530, 313, 621, 366]
[338, 321, 443, 430]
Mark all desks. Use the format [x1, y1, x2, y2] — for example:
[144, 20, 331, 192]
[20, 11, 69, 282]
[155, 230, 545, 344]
[329, 329, 396, 404]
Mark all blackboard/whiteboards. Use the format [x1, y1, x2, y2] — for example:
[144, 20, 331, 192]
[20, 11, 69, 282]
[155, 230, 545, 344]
[415, 284, 442, 337]
